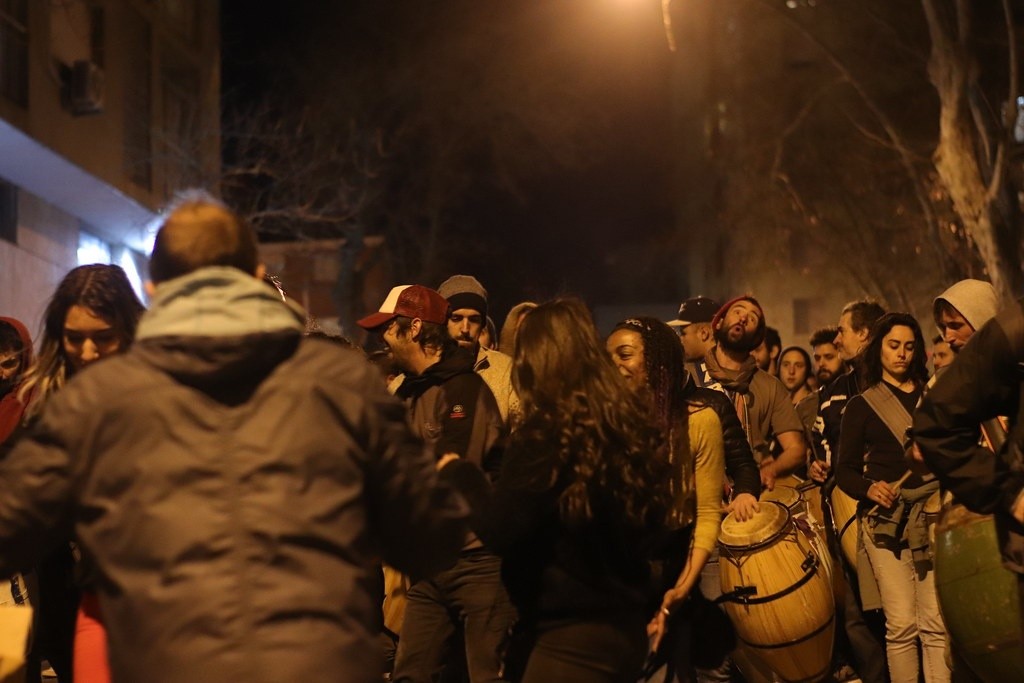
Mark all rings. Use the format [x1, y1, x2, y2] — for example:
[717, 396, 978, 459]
[878, 493, 882, 498]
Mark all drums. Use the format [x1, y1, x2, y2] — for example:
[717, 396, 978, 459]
[925, 492, 953, 539]
[717, 500, 836, 676]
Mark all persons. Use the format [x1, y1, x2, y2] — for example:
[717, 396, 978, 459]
[435, 276, 1024, 683]
[0, 201, 472, 683]
[357, 284, 504, 683]
[439, 296, 669, 683]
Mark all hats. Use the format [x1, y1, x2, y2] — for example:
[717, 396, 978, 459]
[664, 296, 718, 327]
[436, 274, 488, 305]
[356, 285, 447, 330]
